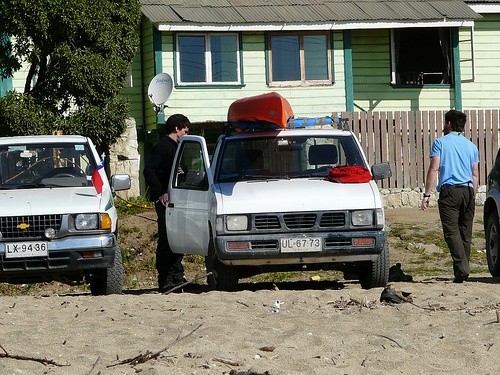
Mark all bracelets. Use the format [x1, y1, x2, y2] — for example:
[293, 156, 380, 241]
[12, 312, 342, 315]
[423, 194, 430, 198]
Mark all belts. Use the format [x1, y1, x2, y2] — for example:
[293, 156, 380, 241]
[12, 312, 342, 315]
[463, 182, 472, 187]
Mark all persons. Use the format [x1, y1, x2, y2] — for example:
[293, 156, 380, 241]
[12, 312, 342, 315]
[421, 111, 479, 283]
[142, 115, 190, 292]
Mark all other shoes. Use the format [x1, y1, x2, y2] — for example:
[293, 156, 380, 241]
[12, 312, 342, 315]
[454, 273, 468, 283]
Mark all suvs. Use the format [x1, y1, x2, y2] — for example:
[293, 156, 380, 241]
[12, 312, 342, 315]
[165, 115, 392, 293]
[0, 135, 131, 296]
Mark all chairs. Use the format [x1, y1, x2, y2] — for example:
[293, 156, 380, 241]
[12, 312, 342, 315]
[235, 149, 265, 172]
[308, 144, 339, 170]
